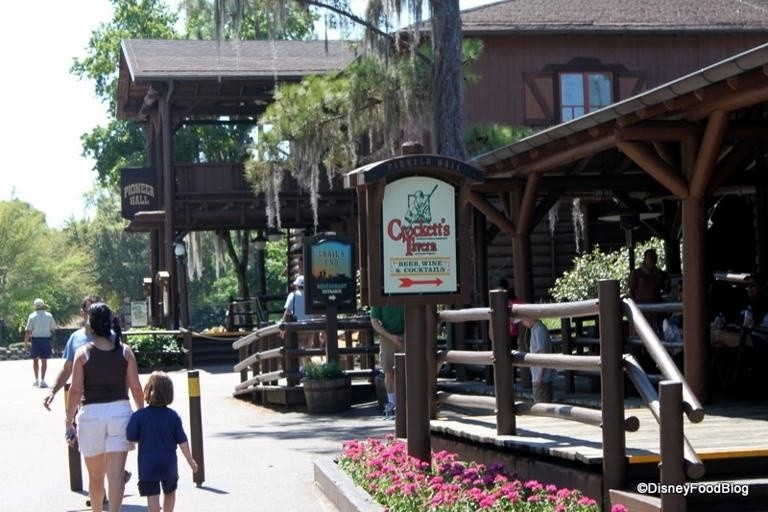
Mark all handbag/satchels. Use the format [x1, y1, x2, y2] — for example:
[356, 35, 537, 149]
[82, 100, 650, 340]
[292, 314, 298, 322]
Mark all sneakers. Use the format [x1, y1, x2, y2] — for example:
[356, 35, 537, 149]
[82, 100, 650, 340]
[383, 402, 395, 416]
[32, 381, 48, 388]
[85, 488, 108, 506]
[124, 470, 132, 484]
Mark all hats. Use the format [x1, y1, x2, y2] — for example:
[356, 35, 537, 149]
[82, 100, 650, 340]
[293, 275, 304, 287]
[34, 298, 44, 307]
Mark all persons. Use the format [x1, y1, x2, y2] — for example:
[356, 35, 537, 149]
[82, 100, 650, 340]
[652, 265, 767, 403]
[64, 301, 145, 511]
[337, 313, 359, 370]
[21, 297, 56, 388]
[487, 277, 519, 387]
[124, 369, 198, 511]
[627, 248, 670, 342]
[278, 275, 327, 370]
[512, 313, 555, 401]
[368, 302, 407, 421]
[225, 301, 241, 332]
[43, 294, 132, 506]
[405, 190, 432, 224]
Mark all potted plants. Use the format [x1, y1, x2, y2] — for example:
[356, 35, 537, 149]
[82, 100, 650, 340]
[304, 360, 352, 414]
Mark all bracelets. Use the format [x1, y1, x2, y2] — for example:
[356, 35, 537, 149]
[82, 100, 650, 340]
[65, 418, 74, 425]
